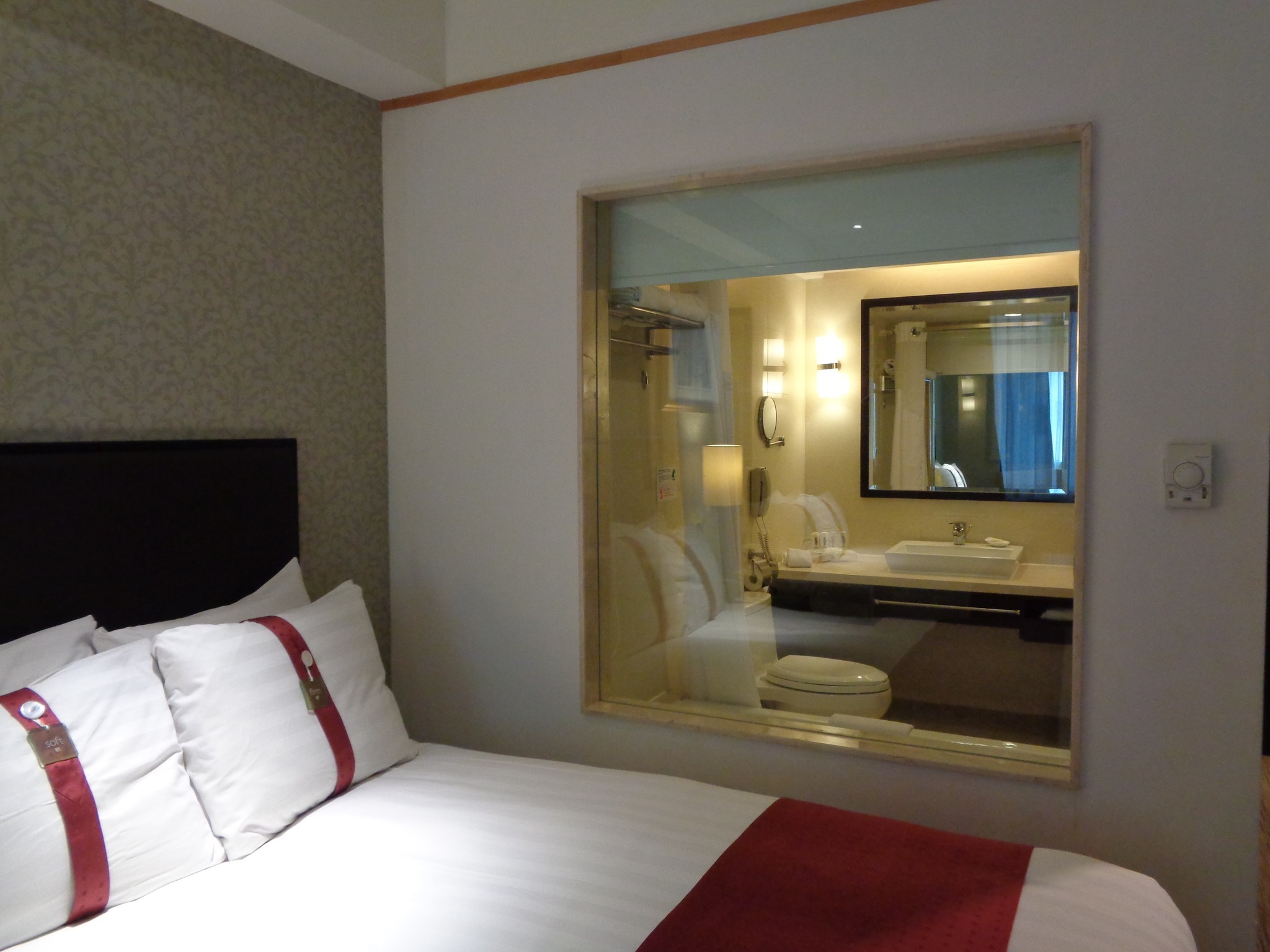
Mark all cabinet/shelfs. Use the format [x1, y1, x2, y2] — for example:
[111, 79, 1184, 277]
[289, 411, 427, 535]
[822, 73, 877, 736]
[607, 303, 706, 360]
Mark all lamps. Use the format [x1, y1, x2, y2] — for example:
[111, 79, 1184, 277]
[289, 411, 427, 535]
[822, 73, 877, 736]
[702, 443, 744, 506]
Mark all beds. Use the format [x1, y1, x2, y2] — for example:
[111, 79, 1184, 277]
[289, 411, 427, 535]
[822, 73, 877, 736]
[0, 437, 1201, 952]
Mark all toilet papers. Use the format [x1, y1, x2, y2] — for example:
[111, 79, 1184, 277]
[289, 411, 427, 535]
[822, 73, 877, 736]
[743, 559, 774, 590]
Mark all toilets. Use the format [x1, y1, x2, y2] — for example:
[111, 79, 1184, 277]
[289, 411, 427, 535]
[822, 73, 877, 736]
[735, 590, 894, 718]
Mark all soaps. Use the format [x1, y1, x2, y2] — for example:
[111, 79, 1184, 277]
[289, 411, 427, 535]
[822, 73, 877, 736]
[985, 537, 1004, 544]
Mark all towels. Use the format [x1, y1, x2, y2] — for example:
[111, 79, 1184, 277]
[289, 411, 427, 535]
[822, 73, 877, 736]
[610, 285, 672, 332]
[885, 359, 936, 380]
[782, 547, 813, 567]
[664, 289, 710, 324]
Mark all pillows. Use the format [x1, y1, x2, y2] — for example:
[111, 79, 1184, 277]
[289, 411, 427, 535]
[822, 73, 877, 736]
[1, 614, 99, 695]
[154, 579, 422, 862]
[934, 460, 968, 488]
[1, 634, 228, 948]
[90, 556, 315, 654]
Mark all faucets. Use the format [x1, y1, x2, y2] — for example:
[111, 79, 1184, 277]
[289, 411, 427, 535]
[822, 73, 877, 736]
[947, 521, 973, 545]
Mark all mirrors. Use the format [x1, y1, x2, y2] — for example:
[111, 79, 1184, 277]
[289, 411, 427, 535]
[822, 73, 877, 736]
[857, 283, 1078, 502]
[760, 394, 786, 447]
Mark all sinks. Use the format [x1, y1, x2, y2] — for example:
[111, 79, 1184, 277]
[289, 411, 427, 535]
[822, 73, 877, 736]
[886, 540, 1024, 580]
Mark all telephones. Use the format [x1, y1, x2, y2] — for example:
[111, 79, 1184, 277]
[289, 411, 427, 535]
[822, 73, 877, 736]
[751, 467, 772, 519]
